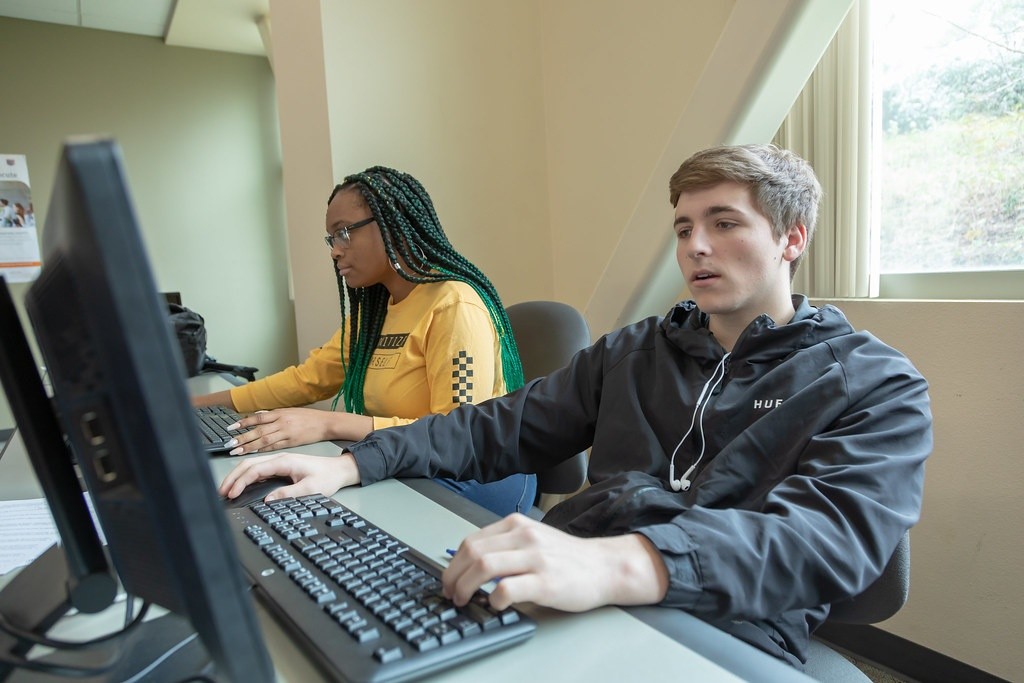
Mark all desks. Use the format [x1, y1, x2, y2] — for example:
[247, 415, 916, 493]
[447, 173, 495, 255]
[1, 350, 820, 681]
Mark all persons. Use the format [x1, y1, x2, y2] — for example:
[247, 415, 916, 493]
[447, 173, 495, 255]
[0, 199, 33, 228]
[192, 165, 537, 517]
[222, 143, 934, 672]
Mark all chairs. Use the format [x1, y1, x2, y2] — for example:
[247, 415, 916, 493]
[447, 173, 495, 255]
[505, 299, 593, 509]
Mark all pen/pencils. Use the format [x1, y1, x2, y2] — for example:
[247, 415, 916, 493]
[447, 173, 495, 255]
[445, 548, 501, 586]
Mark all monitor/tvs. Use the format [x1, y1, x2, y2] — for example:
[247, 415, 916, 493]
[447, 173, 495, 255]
[23, 135, 274, 683]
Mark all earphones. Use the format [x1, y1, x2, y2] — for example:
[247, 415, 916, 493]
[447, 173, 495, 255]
[680, 465, 696, 491]
[669, 465, 681, 491]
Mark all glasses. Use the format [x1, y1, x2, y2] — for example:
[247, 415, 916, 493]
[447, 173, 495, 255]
[324, 217, 375, 250]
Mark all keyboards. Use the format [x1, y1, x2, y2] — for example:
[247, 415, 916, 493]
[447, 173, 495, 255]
[225, 492, 537, 683]
[192, 404, 258, 452]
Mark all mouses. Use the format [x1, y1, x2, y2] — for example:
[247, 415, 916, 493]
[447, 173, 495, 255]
[225, 476, 293, 509]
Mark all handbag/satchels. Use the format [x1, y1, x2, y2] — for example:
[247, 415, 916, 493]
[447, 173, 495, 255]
[169, 303, 209, 375]
